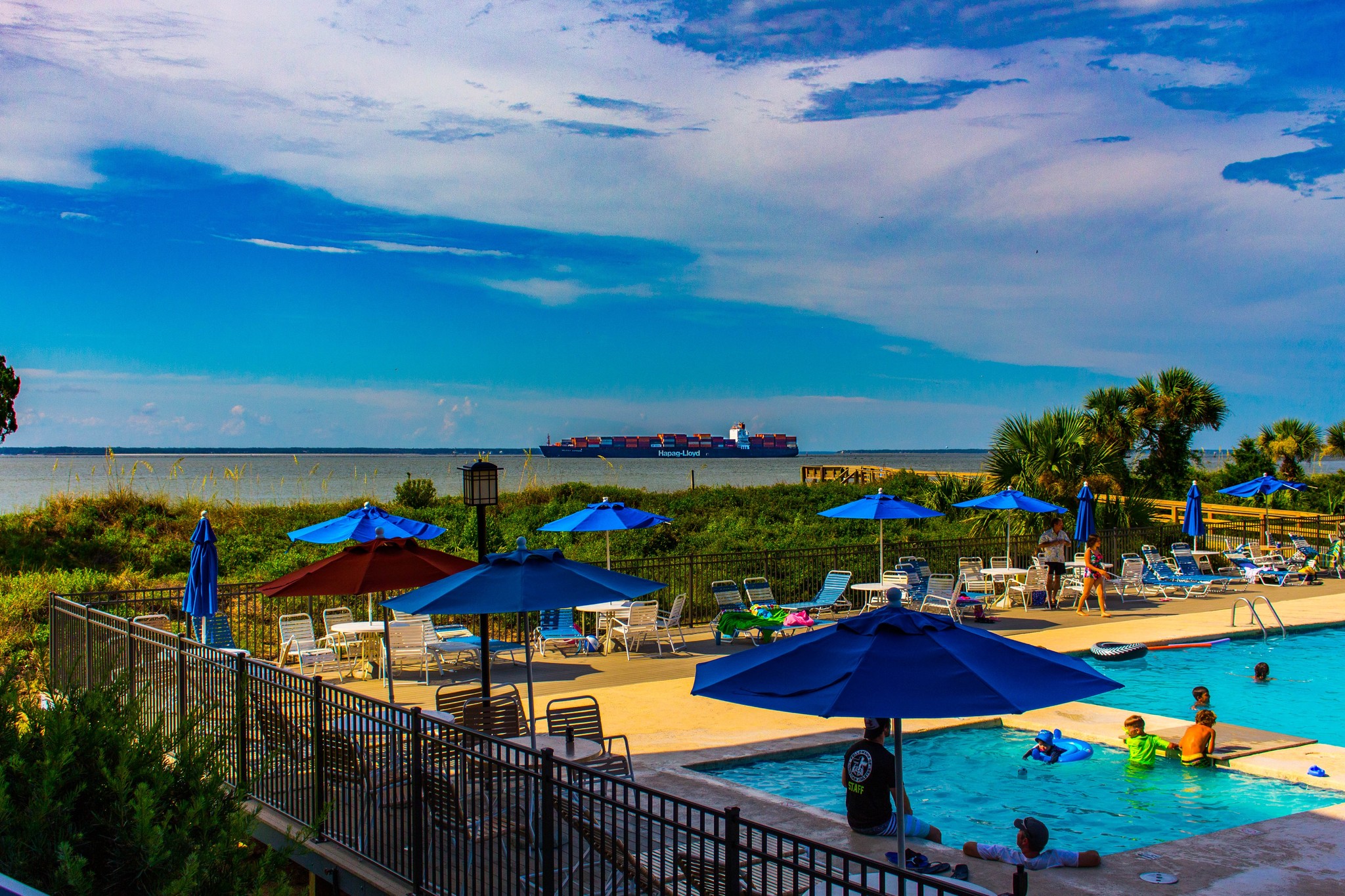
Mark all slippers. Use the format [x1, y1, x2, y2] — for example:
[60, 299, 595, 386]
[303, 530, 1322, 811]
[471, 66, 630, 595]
[1045, 600, 1052, 604]
[885, 848, 928, 861]
[950, 864, 968, 881]
[889, 856, 931, 869]
[989, 616, 1001, 621]
[1052, 600, 1061, 604]
[914, 862, 950, 874]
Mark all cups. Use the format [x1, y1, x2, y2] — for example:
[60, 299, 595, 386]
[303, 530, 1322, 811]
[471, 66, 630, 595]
[715, 631, 721, 646]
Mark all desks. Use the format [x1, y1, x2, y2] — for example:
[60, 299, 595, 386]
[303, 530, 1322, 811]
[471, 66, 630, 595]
[576, 599, 644, 655]
[802, 871, 998, 896]
[330, 621, 409, 679]
[465, 735, 603, 851]
[156, 647, 250, 707]
[850, 583, 913, 604]
[1170, 551, 1219, 569]
[1244, 544, 1292, 578]
[1064, 561, 1113, 600]
[979, 568, 1036, 608]
[332, 709, 455, 809]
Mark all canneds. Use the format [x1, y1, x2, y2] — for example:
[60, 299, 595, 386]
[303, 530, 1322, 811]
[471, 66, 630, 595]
[1277, 541, 1281, 548]
[715, 631, 721, 645]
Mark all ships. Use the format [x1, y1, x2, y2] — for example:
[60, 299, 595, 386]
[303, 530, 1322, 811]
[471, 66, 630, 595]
[539, 421, 799, 458]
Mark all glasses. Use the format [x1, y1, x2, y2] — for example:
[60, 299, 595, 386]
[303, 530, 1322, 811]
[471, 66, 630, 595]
[1022, 816, 1036, 832]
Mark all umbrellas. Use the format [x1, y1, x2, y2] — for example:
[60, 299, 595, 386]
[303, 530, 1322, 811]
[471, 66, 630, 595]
[536, 497, 675, 619]
[817, 488, 946, 604]
[284, 502, 449, 662]
[256, 527, 480, 768]
[182, 510, 218, 644]
[1182, 480, 1205, 551]
[377, 537, 668, 812]
[690, 587, 1127, 896]
[1216, 473, 1318, 559]
[951, 486, 1068, 581]
[1074, 481, 1096, 552]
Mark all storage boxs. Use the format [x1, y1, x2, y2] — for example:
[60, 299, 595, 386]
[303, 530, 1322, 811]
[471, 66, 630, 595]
[556, 434, 798, 450]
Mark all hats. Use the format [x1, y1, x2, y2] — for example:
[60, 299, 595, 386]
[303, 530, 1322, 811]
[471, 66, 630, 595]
[1035, 730, 1055, 746]
[1013, 818, 1048, 847]
[864, 717, 889, 739]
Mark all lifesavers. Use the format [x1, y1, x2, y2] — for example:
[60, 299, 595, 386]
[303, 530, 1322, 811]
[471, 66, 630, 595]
[1147, 638, 1231, 651]
[1032, 729, 1094, 763]
[1089, 641, 1148, 661]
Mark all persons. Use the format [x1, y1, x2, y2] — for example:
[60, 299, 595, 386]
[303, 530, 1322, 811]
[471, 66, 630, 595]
[842, 718, 942, 844]
[1123, 715, 1179, 763]
[1038, 518, 1071, 604]
[1189, 686, 1210, 711]
[963, 817, 1100, 870]
[1249, 662, 1278, 680]
[1178, 709, 1216, 768]
[1076, 534, 1111, 617]
[1022, 730, 1067, 764]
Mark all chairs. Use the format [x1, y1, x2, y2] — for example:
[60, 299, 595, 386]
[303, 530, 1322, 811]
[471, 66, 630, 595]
[112, 569, 853, 896]
[866, 522, 1345, 627]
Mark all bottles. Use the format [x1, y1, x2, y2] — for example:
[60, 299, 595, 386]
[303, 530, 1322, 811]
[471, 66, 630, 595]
[1276, 540, 1281, 548]
[1013, 864, 1028, 896]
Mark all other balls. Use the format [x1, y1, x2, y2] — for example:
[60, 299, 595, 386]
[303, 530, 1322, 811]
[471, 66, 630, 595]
[560, 622, 581, 644]
[584, 636, 599, 652]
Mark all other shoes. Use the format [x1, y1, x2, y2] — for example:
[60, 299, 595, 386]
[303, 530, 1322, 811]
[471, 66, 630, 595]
[1307, 581, 1323, 585]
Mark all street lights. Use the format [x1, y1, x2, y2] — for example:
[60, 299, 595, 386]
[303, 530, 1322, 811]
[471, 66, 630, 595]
[457, 459, 503, 792]
